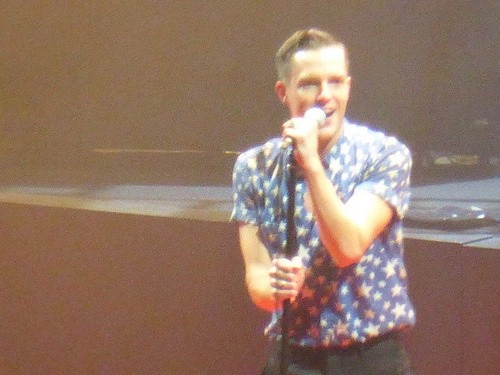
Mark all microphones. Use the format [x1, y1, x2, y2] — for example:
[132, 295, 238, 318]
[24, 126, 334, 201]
[280, 108, 326, 149]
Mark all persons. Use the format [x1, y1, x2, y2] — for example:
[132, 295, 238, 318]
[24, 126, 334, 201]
[228, 27, 420, 375]
